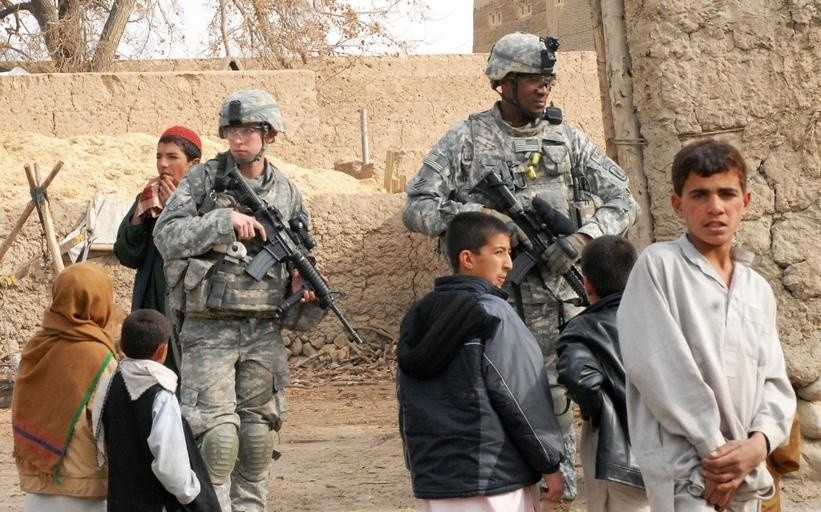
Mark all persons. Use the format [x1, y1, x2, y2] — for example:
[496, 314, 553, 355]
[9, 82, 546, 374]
[113, 125, 202, 377]
[401, 30, 640, 498]
[617, 139, 797, 512]
[92, 309, 223, 512]
[551, 234, 653, 512]
[11, 261, 120, 512]
[152, 89, 329, 512]
[394, 211, 565, 512]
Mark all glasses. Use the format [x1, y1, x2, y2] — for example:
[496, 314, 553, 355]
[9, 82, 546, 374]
[222, 127, 262, 139]
[518, 72, 557, 89]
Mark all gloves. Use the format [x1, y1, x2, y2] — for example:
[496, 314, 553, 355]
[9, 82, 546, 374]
[488, 208, 534, 252]
[540, 233, 585, 276]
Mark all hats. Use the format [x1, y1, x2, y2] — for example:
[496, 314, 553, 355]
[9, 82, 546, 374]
[160, 126, 203, 153]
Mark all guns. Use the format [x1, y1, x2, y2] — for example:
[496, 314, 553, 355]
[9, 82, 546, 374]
[467, 169, 588, 306]
[227, 166, 364, 345]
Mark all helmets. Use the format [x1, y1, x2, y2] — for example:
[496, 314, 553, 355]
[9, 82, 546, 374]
[218, 89, 285, 139]
[484, 32, 556, 81]
